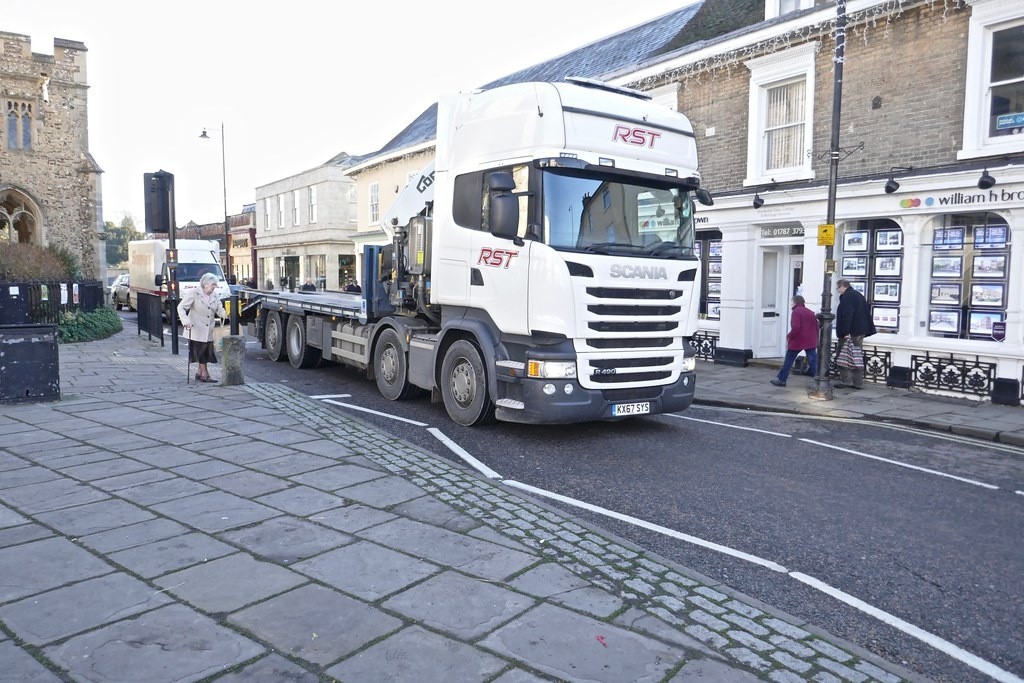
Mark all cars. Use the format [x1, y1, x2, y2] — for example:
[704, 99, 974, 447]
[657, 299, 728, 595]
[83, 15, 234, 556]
[110, 274, 130, 310]
[126, 289, 137, 312]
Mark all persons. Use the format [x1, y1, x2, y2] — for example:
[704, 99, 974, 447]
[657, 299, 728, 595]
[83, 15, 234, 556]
[302, 278, 316, 291]
[769, 295, 819, 387]
[348, 280, 361, 292]
[177, 273, 230, 383]
[834, 280, 877, 389]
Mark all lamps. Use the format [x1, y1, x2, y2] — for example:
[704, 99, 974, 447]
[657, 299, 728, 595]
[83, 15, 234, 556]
[885, 166, 913, 193]
[977, 155, 1009, 189]
[753, 183, 778, 209]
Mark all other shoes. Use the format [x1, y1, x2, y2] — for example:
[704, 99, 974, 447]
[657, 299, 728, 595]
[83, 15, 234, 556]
[200, 377, 218, 383]
[834, 383, 848, 388]
[848, 385, 863, 389]
[770, 379, 787, 386]
[196, 373, 201, 379]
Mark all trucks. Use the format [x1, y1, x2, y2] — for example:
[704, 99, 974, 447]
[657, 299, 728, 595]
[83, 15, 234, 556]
[127, 240, 230, 325]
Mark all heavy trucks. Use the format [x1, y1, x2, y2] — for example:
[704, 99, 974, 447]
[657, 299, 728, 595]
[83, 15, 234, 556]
[228, 79, 722, 429]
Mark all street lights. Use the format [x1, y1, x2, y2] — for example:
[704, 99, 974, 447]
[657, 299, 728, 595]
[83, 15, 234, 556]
[199, 76, 249, 386]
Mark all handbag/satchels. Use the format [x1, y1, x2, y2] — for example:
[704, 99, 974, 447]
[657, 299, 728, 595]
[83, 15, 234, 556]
[836, 335, 864, 369]
